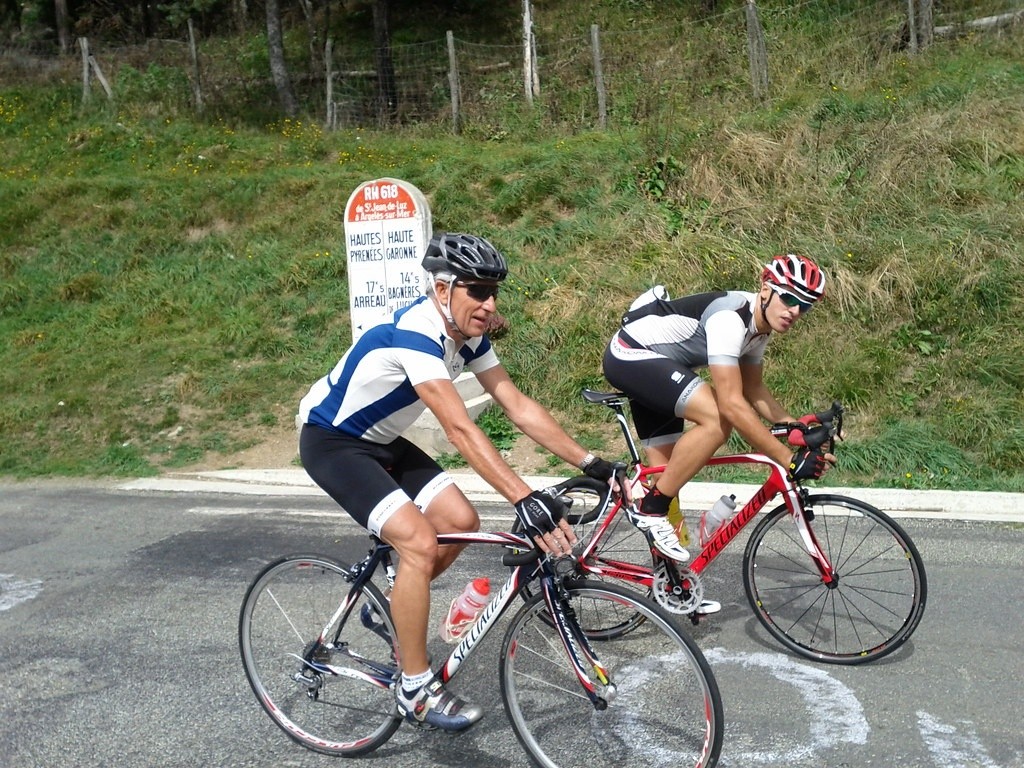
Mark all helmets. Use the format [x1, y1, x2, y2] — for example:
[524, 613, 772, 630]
[762, 254, 826, 305]
[421, 232, 508, 281]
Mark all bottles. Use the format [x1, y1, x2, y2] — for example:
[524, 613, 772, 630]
[668, 496, 690, 547]
[438, 578, 491, 645]
[694, 494, 737, 539]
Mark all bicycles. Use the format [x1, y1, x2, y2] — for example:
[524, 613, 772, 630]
[235, 473, 728, 768]
[508, 387, 930, 666]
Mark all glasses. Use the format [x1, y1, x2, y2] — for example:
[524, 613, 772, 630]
[764, 281, 813, 313]
[451, 280, 498, 302]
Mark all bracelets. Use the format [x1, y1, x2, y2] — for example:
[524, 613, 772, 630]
[581, 454, 594, 470]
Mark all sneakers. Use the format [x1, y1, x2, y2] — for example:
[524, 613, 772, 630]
[395, 674, 485, 730]
[652, 590, 721, 617]
[360, 602, 432, 666]
[625, 497, 690, 563]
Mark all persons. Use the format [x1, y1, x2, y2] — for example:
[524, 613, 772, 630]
[602, 255, 838, 616]
[294, 232, 634, 737]
